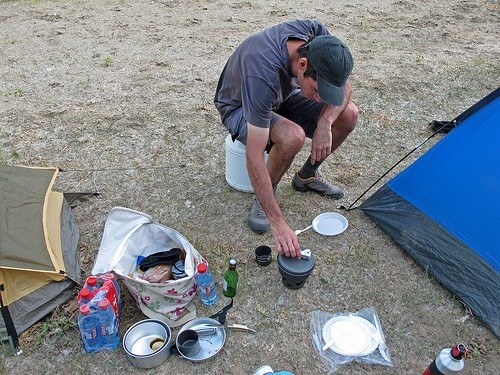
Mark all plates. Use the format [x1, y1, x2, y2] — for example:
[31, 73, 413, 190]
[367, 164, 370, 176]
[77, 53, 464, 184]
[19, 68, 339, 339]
[176, 317, 226, 363]
[312, 211, 348, 236]
[323, 315, 381, 356]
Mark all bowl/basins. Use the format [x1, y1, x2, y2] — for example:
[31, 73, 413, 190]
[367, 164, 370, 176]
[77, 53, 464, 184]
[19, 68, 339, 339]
[123, 318, 176, 368]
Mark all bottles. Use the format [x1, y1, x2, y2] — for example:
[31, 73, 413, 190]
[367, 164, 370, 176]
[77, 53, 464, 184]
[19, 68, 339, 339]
[77, 273, 121, 352]
[196, 263, 218, 305]
[422, 343, 466, 375]
[222, 258, 239, 297]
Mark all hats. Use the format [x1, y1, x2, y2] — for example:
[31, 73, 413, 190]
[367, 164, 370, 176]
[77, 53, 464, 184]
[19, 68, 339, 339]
[308, 36, 354, 106]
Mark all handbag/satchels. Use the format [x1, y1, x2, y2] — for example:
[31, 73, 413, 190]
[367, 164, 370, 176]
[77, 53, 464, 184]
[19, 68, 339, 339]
[90, 207, 209, 329]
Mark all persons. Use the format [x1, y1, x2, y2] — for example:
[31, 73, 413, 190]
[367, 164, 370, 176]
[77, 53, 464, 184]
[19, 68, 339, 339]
[213, 19, 362, 260]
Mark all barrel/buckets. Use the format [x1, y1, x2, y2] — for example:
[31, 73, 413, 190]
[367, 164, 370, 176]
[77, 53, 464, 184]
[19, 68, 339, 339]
[225, 132, 269, 193]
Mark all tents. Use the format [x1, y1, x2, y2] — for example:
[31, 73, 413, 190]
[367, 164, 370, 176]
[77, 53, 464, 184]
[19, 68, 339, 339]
[343, 84, 500, 340]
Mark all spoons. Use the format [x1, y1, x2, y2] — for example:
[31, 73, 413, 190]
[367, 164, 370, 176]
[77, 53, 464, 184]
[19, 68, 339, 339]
[295, 225, 312, 235]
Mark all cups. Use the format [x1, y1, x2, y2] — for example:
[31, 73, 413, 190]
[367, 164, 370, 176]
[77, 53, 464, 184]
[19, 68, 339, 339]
[277, 247, 315, 289]
[255, 246, 272, 266]
[177, 329, 200, 356]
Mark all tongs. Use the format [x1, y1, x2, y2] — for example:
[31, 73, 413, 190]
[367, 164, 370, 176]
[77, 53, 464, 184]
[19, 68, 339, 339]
[189, 324, 256, 333]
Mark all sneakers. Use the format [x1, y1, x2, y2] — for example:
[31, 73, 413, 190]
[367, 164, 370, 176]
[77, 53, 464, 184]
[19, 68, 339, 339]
[248, 187, 276, 233]
[290, 170, 345, 200]
